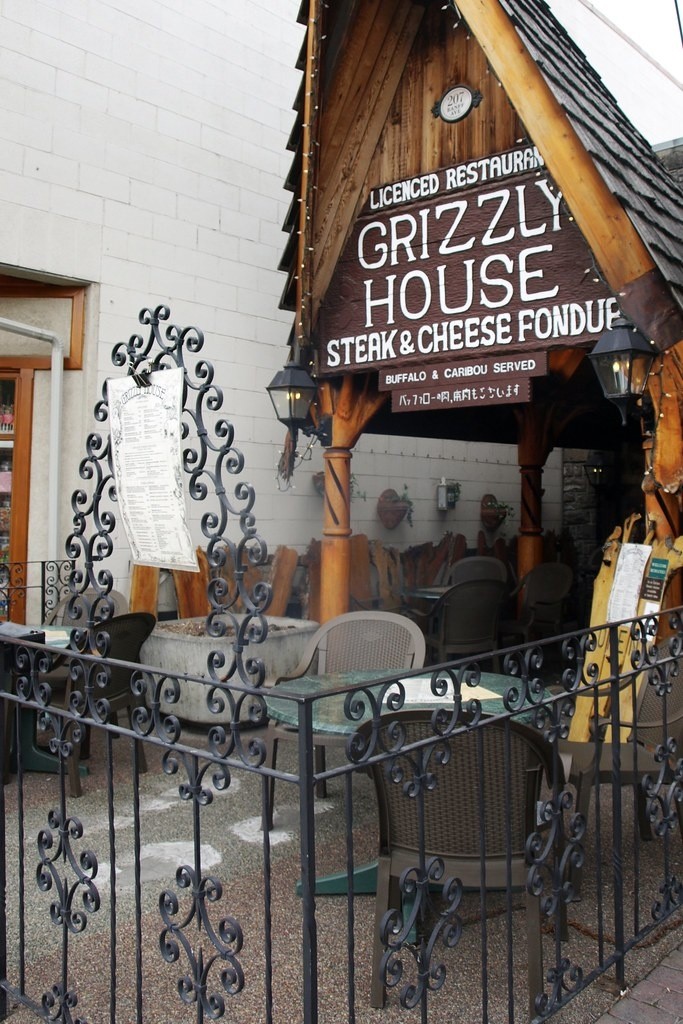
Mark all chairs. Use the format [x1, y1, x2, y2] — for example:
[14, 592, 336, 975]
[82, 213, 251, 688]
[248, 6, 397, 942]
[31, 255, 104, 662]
[428, 555, 507, 635]
[350, 594, 421, 627]
[354, 708, 567, 1024]
[499, 561, 573, 677]
[423, 578, 510, 674]
[0, 613, 156, 799]
[260, 611, 426, 832]
[557, 637, 683, 904]
[43, 588, 128, 737]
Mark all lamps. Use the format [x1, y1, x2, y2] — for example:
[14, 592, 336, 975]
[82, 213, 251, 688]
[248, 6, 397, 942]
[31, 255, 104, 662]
[585, 319, 657, 434]
[582, 451, 613, 491]
[264, 360, 332, 447]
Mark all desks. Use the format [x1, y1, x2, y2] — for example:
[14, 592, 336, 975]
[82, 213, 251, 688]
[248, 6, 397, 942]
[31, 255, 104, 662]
[0, 624, 90, 777]
[265, 669, 554, 946]
[402, 584, 453, 662]
